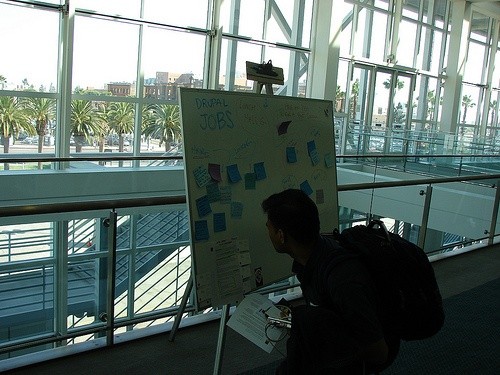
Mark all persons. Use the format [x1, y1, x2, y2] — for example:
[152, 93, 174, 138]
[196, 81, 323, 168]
[261, 189, 401, 375]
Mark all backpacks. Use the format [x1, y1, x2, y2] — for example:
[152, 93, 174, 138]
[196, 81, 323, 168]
[322, 219, 446, 342]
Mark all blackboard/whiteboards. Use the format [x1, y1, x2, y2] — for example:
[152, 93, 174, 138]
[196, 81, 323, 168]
[179, 88, 341, 310]
